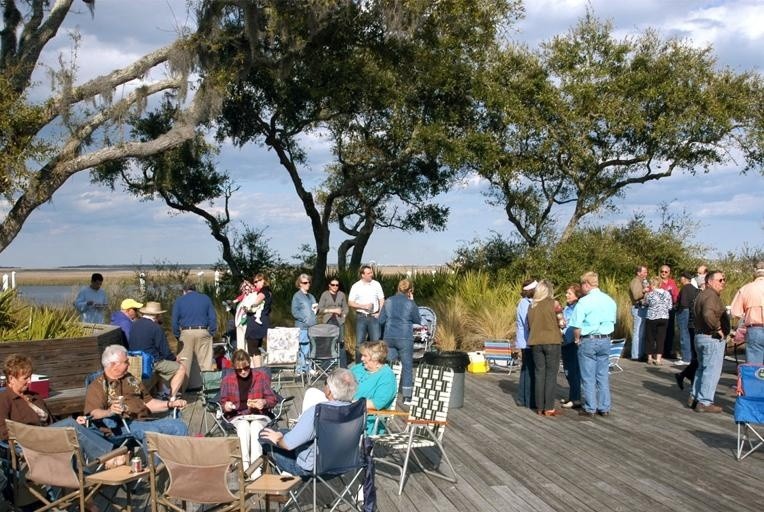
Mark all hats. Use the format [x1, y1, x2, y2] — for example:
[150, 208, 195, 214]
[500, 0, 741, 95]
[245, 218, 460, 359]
[120, 298, 144, 310]
[139, 301, 169, 315]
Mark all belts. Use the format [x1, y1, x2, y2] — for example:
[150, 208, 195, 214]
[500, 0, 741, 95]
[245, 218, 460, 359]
[356, 310, 380, 317]
[579, 334, 613, 339]
[746, 323, 764, 328]
[182, 326, 207, 330]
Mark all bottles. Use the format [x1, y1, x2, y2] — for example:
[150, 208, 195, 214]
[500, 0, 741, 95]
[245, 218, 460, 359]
[739, 311, 746, 329]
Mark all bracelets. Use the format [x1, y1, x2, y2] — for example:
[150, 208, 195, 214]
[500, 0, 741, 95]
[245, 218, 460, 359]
[732, 326, 738, 331]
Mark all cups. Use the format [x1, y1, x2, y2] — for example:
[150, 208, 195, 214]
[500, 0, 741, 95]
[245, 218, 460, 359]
[0, 375, 6, 387]
[114, 396, 125, 409]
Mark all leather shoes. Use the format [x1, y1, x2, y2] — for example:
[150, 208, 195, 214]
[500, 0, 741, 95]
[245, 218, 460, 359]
[578, 408, 609, 417]
[537, 408, 563, 416]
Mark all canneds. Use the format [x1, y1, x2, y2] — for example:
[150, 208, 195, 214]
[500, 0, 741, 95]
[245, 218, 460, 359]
[115, 396, 125, 411]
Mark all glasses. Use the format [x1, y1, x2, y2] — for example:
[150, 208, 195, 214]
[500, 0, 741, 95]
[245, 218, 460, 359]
[300, 281, 311, 284]
[712, 278, 725, 282]
[252, 278, 263, 284]
[330, 283, 339, 286]
[660, 270, 670, 273]
[234, 361, 250, 372]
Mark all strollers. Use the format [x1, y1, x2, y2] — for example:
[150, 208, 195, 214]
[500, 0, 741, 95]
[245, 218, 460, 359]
[409, 305, 440, 366]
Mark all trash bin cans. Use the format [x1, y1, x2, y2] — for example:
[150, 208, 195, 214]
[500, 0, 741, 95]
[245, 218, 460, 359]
[424, 351, 465, 408]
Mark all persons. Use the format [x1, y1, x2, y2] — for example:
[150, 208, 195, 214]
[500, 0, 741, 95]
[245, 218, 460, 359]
[730, 261, 764, 364]
[0, 354, 132, 511]
[515, 263, 731, 418]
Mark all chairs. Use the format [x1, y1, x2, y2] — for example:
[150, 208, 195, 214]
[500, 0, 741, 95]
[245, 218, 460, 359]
[732, 362, 763, 462]
[411, 307, 437, 366]
[482, 338, 522, 376]
[608, 336, 627, 375]
[367, 363, 458, 495]
[2, 323, 410, 512]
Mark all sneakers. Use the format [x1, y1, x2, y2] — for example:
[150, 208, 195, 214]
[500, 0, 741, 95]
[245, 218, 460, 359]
[647, 358, 665, 366]
[674, 373, 684, 390]
[560, 396, 582, 409]
[687, 396, 723, 414]
